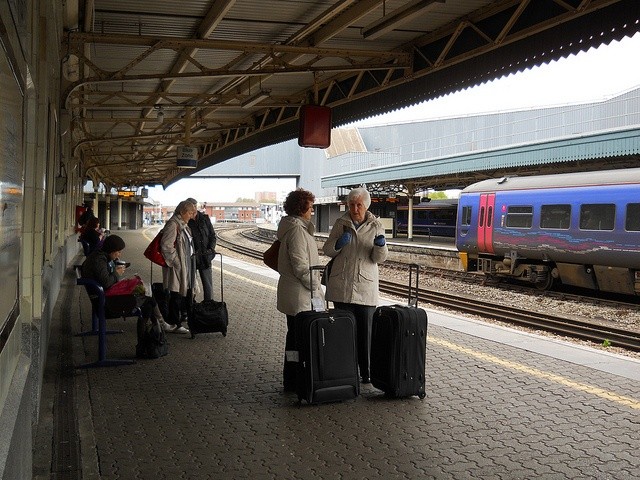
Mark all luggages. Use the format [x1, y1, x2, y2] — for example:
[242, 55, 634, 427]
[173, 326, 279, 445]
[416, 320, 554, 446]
[293, 264, 361, 406]
[369, 263, 428, 401]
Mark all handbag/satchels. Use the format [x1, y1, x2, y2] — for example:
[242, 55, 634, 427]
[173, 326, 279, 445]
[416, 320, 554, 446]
[136, 306, 170, 359]
[188, 298, 228, 334]
[143, 219, 180, 268]
[320, 225, 347, 286]
[262, 239, 281, 271]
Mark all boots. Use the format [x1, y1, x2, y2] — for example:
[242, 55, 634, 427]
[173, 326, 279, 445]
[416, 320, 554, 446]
[152, 304, 177, 332]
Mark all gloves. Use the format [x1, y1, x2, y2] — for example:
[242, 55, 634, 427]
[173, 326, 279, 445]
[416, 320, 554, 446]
[337, 231, 351, 247]
[374, 234, 385, 247]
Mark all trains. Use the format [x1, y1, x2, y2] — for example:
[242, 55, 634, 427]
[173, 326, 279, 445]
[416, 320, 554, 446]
[365, 197, 456, 241]
[454, 167, 639, 301]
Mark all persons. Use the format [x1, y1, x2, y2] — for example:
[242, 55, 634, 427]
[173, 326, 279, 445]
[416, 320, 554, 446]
[321, 187, 390, 385]
[275, 189, 329, 394]
[81, 217, 131, 269]
[185, 197, 217, 303]
[78, 208, 95, 226]
[159, 200, 198, 336]
[82, 234, 178, 335]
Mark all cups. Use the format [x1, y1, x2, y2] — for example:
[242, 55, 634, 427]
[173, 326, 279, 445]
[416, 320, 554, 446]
[116, 263, 127, 274]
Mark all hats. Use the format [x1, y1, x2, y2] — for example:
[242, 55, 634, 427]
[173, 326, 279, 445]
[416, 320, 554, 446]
[102, 234, 126, 253]
[187, 197, 198, 204]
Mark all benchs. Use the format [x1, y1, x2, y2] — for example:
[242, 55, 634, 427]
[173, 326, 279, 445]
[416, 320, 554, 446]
[72, 263, 136, 368]
[77, 236, 101, 257]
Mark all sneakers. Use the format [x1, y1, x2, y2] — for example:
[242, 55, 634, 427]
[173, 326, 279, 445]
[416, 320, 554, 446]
[174, 326, 189, 334]
